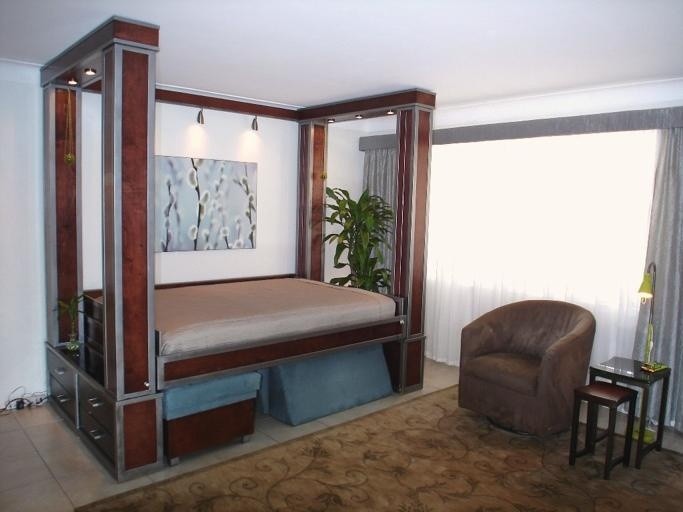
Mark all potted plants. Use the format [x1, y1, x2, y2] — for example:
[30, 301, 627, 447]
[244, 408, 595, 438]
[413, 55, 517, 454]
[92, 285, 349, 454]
[50, 294, 91, 353]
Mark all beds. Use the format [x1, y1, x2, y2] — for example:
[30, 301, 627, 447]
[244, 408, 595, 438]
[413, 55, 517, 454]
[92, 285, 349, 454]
[40, 16, 437, 478]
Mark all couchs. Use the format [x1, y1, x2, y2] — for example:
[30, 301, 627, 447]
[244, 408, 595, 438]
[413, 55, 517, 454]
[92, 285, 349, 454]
[458, 299, 596, 439]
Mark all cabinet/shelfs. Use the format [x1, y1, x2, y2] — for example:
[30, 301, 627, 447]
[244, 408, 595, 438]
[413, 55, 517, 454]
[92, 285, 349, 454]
[586, 356, 672, 467]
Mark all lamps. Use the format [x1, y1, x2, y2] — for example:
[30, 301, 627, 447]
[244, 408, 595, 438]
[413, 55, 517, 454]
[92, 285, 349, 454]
[195, 106, 261, 133]
[635, 262, 669, 373]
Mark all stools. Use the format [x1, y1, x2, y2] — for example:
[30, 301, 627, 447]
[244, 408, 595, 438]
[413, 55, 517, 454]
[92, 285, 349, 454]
[569, 380, 636, 482]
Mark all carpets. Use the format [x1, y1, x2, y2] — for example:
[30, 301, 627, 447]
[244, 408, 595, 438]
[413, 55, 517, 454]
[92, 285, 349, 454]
[68, 378, 680, 511]
